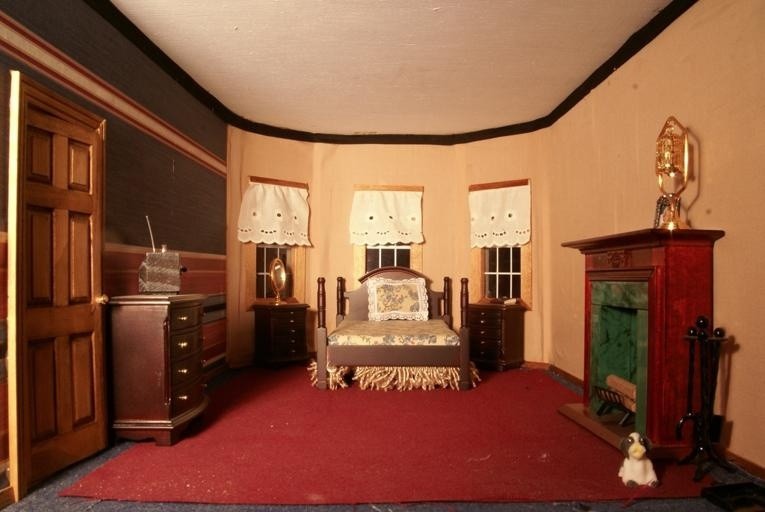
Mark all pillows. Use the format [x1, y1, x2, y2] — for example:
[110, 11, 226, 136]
[364, 271, 430, 324]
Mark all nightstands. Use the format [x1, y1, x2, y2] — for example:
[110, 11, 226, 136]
[247, 299, 312, 372]
[466, 298, 528, 373]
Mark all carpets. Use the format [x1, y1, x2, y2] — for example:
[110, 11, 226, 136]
[55, 353, 721, 509]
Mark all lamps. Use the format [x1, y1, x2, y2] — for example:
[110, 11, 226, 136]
[651, 111, 692, 232]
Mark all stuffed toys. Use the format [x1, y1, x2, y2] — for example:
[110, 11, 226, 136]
[615, 431, 660, 488]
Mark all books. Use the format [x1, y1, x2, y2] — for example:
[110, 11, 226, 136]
[489, 297, 517, 304]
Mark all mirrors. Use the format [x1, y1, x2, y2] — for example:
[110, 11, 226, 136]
[266, 256, 289, 304]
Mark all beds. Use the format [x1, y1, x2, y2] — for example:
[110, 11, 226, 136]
[308, 264, 485, 394]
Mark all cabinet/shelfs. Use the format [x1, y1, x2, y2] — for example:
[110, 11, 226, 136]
[105, 288, 216, 449]
